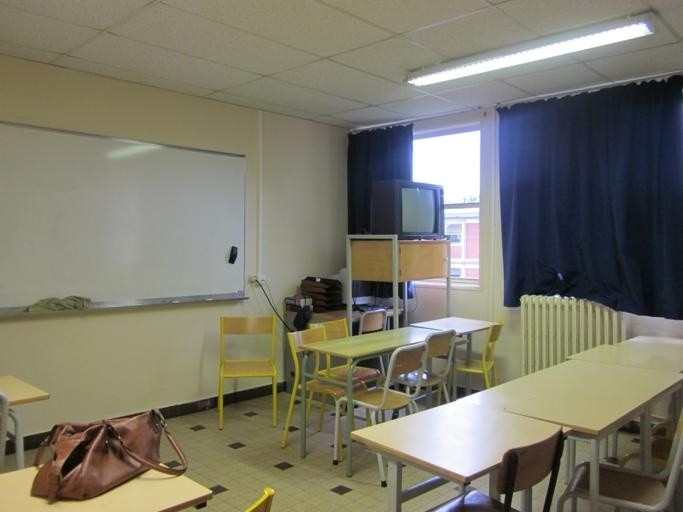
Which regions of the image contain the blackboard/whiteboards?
[0,120,246,318]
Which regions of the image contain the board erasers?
[229,246,237,264]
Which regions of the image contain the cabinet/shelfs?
[345,234,451,334]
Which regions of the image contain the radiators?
[519,294,625,375]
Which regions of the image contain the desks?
[0,374,213,511]
[282,306,409,416]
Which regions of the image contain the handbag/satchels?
[31,407,187,502]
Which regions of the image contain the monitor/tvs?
[368,179,445,240]
